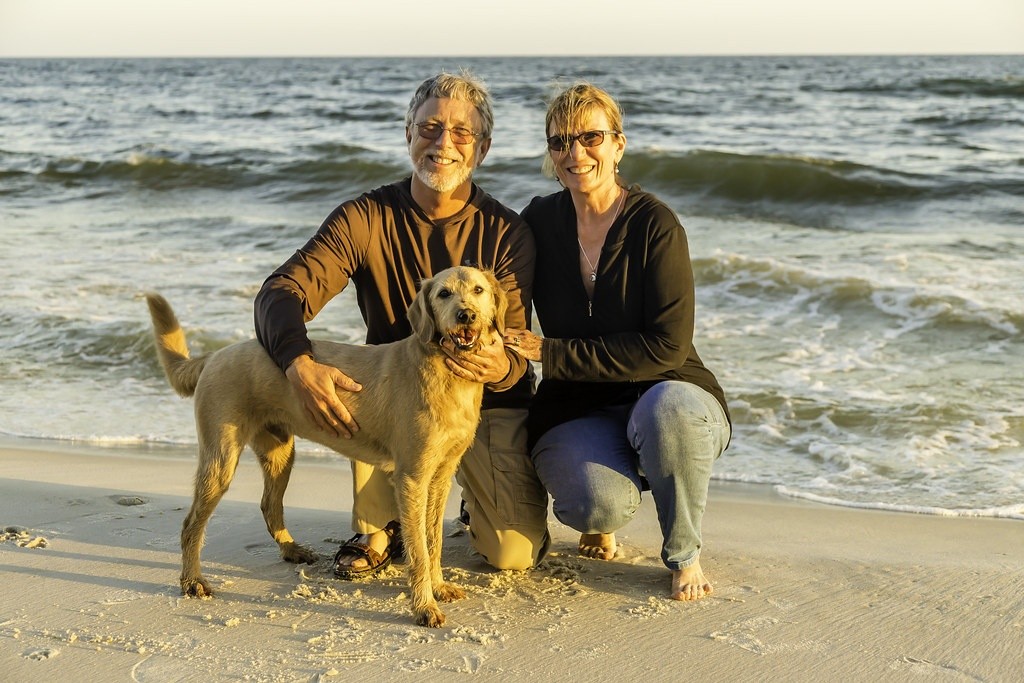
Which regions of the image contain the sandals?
[460,499,470,525]
[335,521,405,580]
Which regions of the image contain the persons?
[254,70,551,579]
[502,83,733,604]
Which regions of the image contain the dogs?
[144,265,509,630]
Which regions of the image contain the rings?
[514,336,520,344]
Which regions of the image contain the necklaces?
[578,189,624,282]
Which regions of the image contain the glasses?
[410,122,483,144]
[547,130,622,152]
